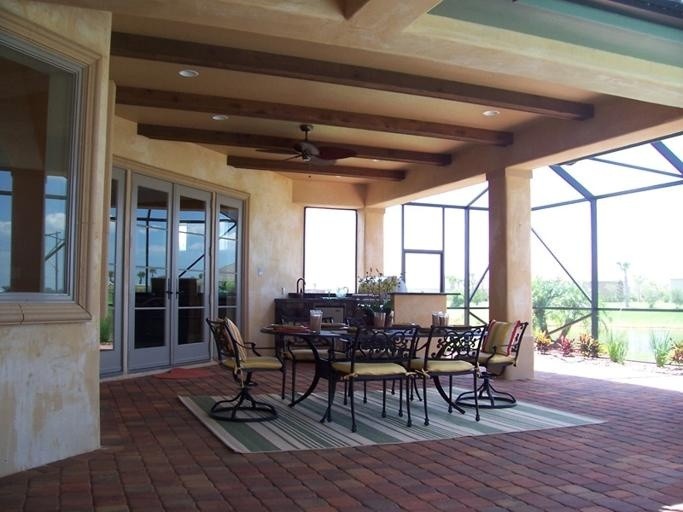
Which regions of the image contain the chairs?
[282,315,349,399]
[344,317,419,404]
[455,319,528,410]
[206,317,289,423]
[319,324,421,432]
[399,324,488,425]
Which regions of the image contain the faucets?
[297,278,305,293]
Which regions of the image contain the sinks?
[288,293,336,298]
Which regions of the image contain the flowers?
[534,329,601,359]
[357,267,406,313]
[668,337,682,367]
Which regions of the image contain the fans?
[255,124,357,163]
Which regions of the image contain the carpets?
[152,368,213,379]
[177,385,608,454]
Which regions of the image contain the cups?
[309,309,324,335]
[336,287,349,298]
